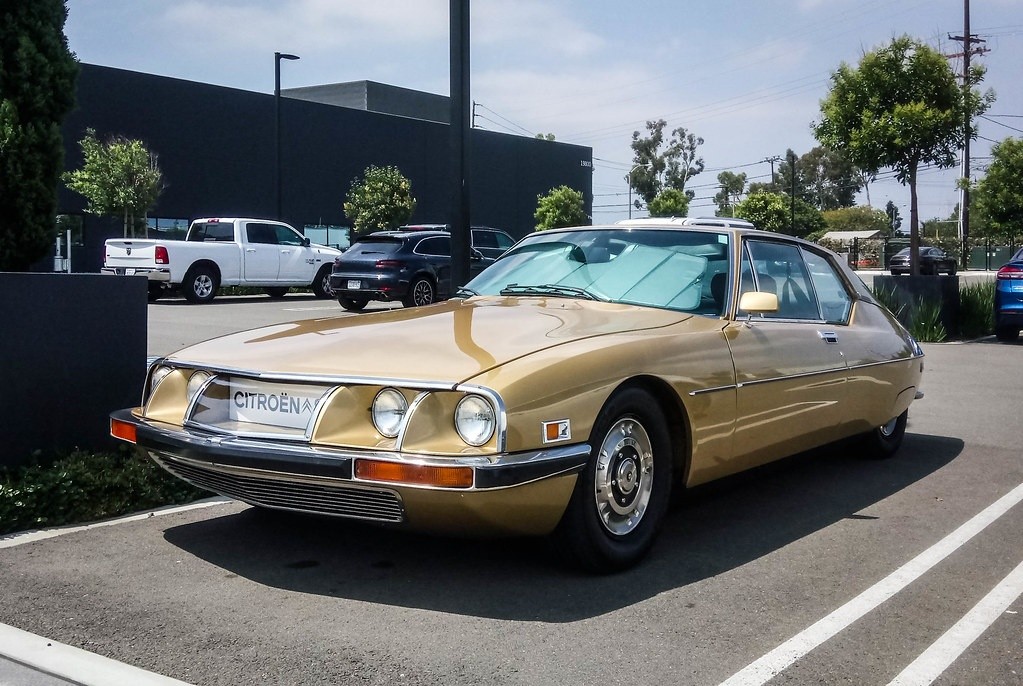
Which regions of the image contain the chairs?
[711,268,780,316]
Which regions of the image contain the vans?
[611,216,756,263]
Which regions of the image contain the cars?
[110,221,928,570]
[888,246,958,275]
[993,248,1023,337]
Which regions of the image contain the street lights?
[273,51,302,218]
[893,204,908,238]
[628,164,650,219]
[719,183,735,218]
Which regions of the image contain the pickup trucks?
[101,217,343,304]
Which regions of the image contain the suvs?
[328,224,495,310]
[468,226,517,258]
